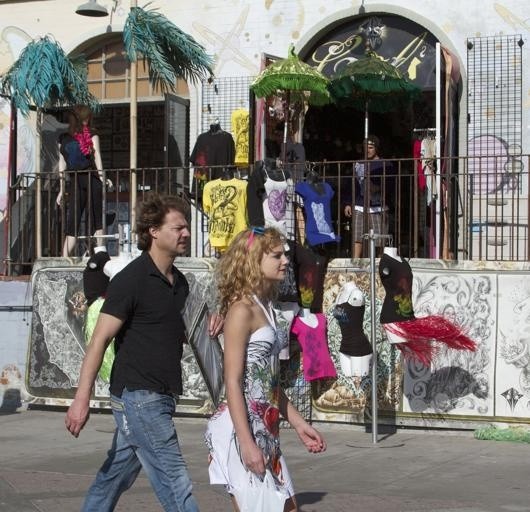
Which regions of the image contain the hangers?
[418,127,436,140]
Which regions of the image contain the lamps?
[75,0,118,17]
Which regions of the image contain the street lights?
[74,0,139,248]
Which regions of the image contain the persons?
[291,307,337,381]
[337,273,351,287]
[64,194,224,512]
[333,281,373,377]
[83,246,115,382]
[439,304,455,323]
[102,252,138,279]
[191,120,235,204]
[58,106,114,258]
[379,246,417,345]
[341,136,394,258]
[203,227,327,512]
